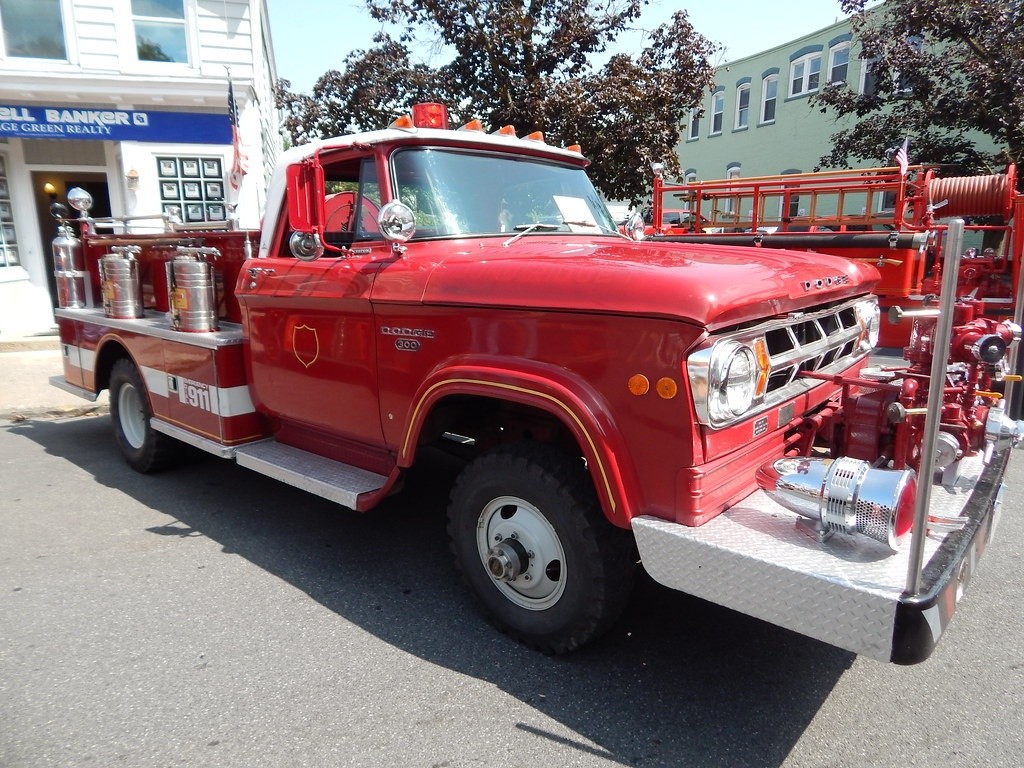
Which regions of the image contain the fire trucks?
[54,101,1024,668]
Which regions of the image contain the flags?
[894,137,910,176]
[227,77,249,192]
